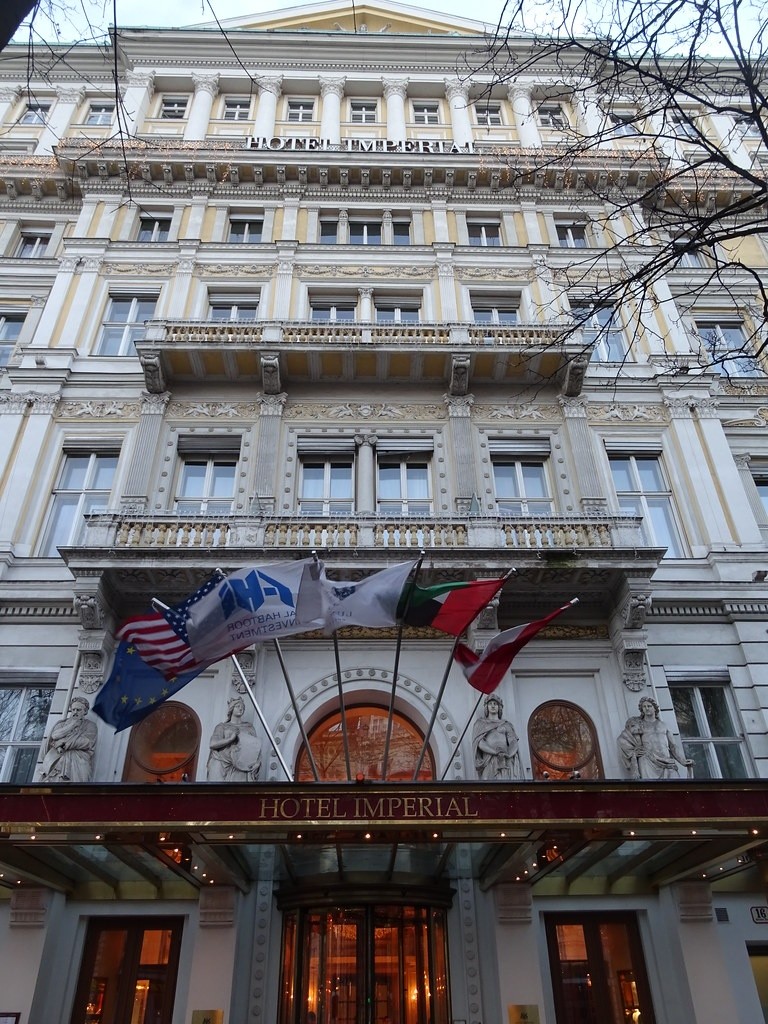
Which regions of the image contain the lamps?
[332,922,392,940]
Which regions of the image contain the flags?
[90,607,209,736]
[295,559,419,635]
[113,574,256,684]
[451,602,572,696]
[397,577,507,638]
[186,557,330,661]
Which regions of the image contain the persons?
[471,693,524,780]
[208,697,261,782]
[617,696,696,778]
[38,696,98,782]
